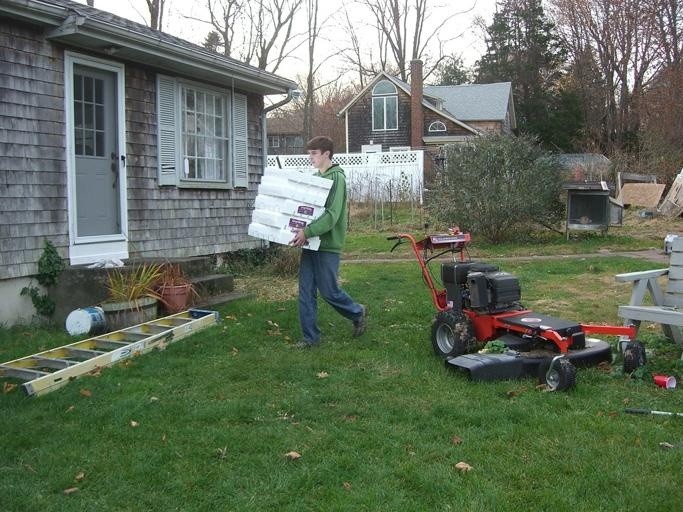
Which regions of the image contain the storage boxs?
[248,165,334,252]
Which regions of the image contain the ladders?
[0,309,219,396]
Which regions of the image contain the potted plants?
[96,257,166,332]
[159,255,204,315]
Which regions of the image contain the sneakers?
[297,340,322,351]
[352,303,366,338]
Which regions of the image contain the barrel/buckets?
[64,306,106,337]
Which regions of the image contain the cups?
[653,375,676,389]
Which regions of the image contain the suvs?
[540,153,612,183]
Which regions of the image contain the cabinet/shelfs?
[560,179,613,242]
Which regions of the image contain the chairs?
[613,234,682,352]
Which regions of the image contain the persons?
[286,137,368,350]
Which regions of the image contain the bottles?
[448,229,454,236]
